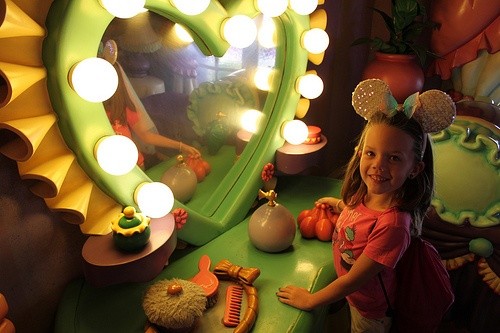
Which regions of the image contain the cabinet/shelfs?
[56,175,346,333]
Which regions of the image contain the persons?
[102,57,202,171]
[275,109,434,333]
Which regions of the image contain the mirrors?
[0,0,329,246]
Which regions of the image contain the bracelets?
[335,200,342,213]
[178,141,182,152]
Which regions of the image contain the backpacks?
[370,207,455,333]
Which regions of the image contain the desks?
[128,76,164,98]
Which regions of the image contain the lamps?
[116,24,162,76]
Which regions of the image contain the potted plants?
[350,0,446,104]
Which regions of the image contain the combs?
[189,254,218,305]
[223,285,243,327]
